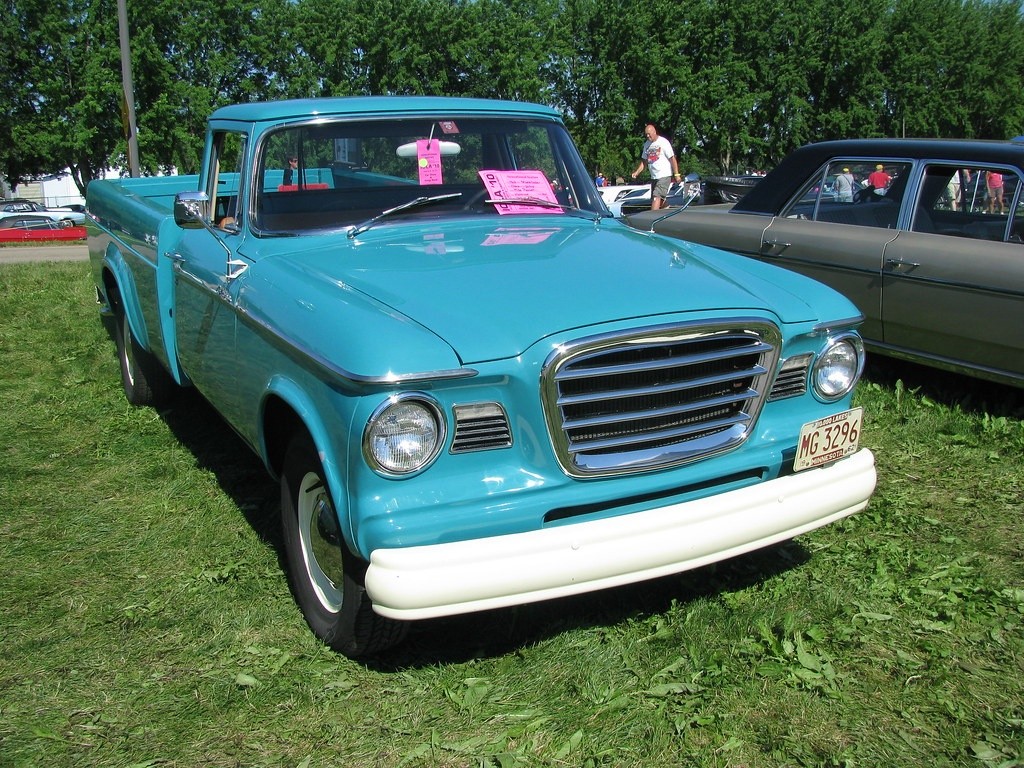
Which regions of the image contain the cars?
[593,180,706,217]
[615,137,1024,400]
[0,197,88,243]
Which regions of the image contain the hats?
[843,167,849,172]
[598,172,602,176]
[876,165,883,170]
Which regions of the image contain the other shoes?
[1000,211,1004,215]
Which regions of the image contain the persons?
[812,164,890,202]
[749,168,767,177]
[596,172,626,187]
[983,171,1005,215]
[553,178,574,206]
[632,124,681,210]
[283,155,298,186]
[945,169,971,211]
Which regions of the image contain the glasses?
[292,160,298,163]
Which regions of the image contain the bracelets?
[673,173,681,177]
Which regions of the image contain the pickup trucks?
[81,93,877,663]
